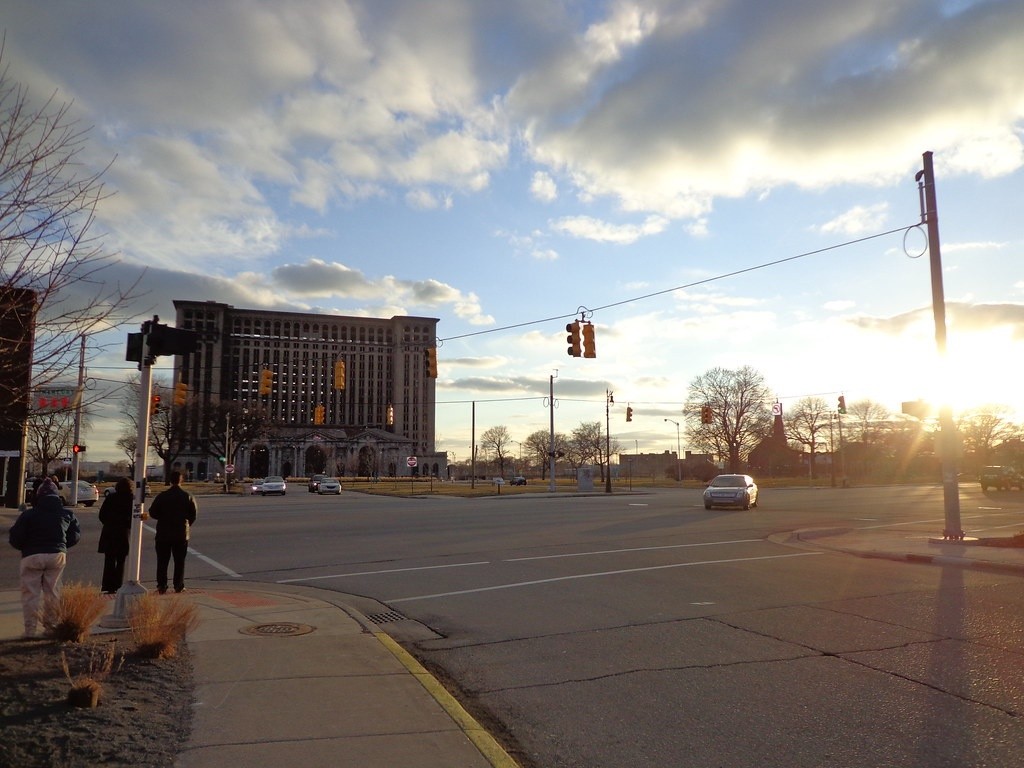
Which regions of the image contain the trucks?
[493,477,505,485]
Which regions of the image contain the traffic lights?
[151,395,161,414]
[73,446,86,452]
[702,407,712,424]
[425,348,437,379]
[566,322,581,357]
[627,407,633,421]
[548,452,554,457]
[838,396,847,414]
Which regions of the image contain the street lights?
[664,418,682,480]
[605,389,615,493]
[511,440,521,459]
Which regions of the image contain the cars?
[249,479,265,495]
[318,478,342,495]
[262,476,287,496]
[25,477,152,507]
[703,474,760,511]
[1013,473,1024,490]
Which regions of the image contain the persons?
[52,475,58,488]
[149,471,197,595]
[98,478,134,593]
[32,475,43,491]
[8,478,80,639]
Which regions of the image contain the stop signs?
[407,457,417,467]
[225,465,234,473]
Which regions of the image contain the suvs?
[980,465,1015,491]
[510,477,527,486]
[308,475,331,493]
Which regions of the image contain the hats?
[37,477,59,496]
